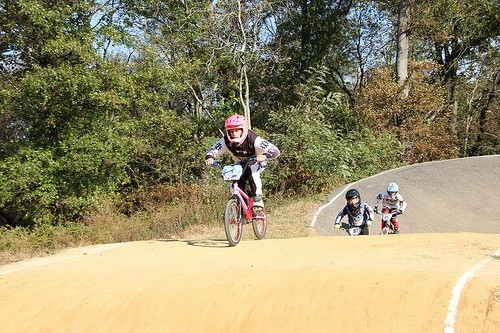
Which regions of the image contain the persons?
[206,114,280,225]
[334,189,374,235]
[374,182,408,234]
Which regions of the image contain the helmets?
[224,114,248,147]
[345,189,361,212]
[387,183,399,199]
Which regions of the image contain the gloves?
[335,224,340,229]
[367,220,372,227]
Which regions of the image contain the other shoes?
[394,228,399,234]
[253,206,263,217]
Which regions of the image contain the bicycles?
[204,156,267,246]
[334,217,374,237]
[376,209,403,236]
